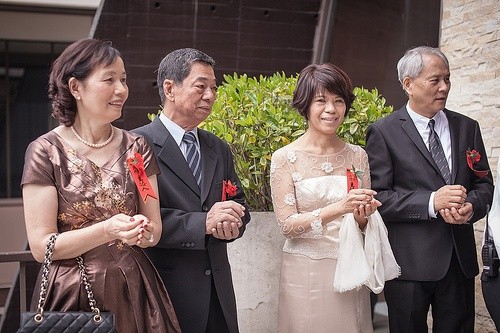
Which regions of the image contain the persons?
[128,47,251,333]
[480,160,500,333]
[270,63,382,333]
[20,39,182,333]
[365,46,495,333]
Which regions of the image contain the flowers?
[288,153,297,163]
[309,219,324,234]
[467,147,481,163]
[225,179,238,197]
[284,193,296,206]
[350,165,365,180]
[312,208,322,218]
[270,159,277,173]
[321,162,334,174]
[291,172,303,182]
[126,152,145,180]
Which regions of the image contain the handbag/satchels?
[19,233,116,332]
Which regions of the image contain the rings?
[147,233,154,242]
[364,216,369,218]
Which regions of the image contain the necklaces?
[70,122,115,148]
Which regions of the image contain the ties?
[182,132,202,189]
[428,119,452,185]
